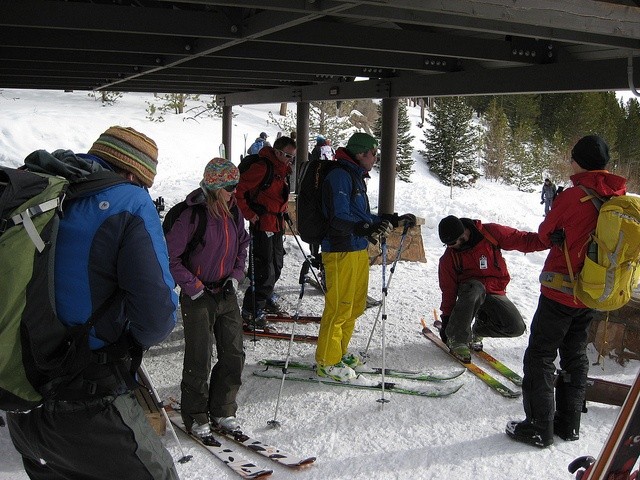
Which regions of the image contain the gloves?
[223,281,236,297]
[383,213,416,230]
[546,229,567,246]
[440,315,449,344]
[194,287,211,303]
[353,219,388,245]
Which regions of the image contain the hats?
[199,157,240,197]
[438,215,464,244]
[87,125,158,187]
[571,135,610,172]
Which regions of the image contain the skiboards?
[242,314,321,343]
[305,269,381,308]
[253,360,465,397]
[163,396,316,478]
[422,312,523,398]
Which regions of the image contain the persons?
[276,131,282,139]
[537,178,561,218]
[289,130,298,139]
[246,132,272,155]
[238,137,299,336]
[437,214,563,363]
[0,124,180,479]
[312,132,417,382]
[167,157,250,440]
[506,134,640,450]
[321,136,337,152]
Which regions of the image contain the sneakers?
[469,332,483,352]
[190,419,212,438]
[316,361,355,383]
[448,339,471,364]
[213,415,240,432]
[343,353,368,372]
[554,423,580,442]
[264,297,285,313]
[241,313,269,328]
[506,421,553,448]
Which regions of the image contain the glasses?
[447,242,457,246]
[223,183,240,192]
[371,151,377,157]
[284,153,295,159]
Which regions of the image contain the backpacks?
[296,159,358,245]
[163,200,207,264]
[1,149,136,414]
[562,186,640,312]
[238,154,290,196]
[251,142,263,154]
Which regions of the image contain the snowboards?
[552,369,631,404]
[569,370,640,480]
